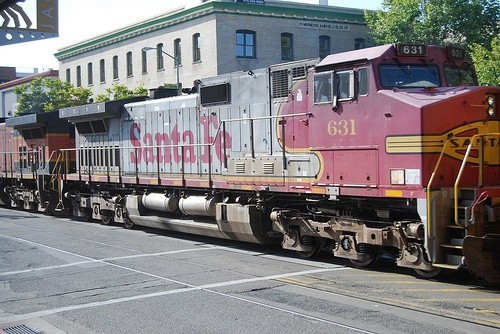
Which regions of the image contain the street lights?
[142,46,179,84]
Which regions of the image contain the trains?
[0,42,500,290]
